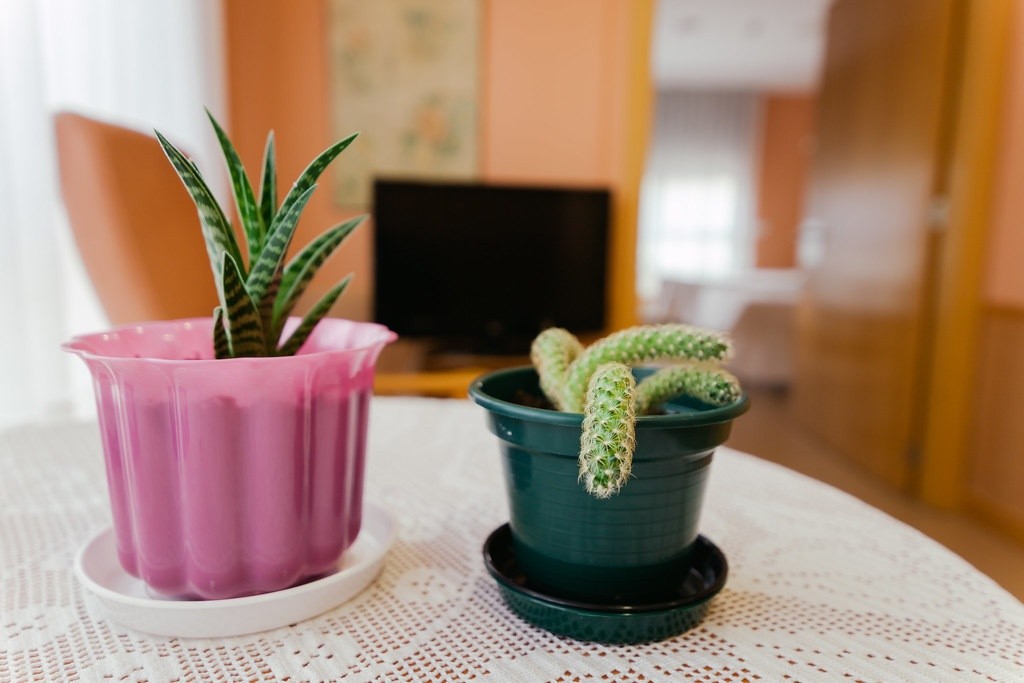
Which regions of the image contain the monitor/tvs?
[369,176,611,339]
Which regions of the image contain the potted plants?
[53,109,405,643]
[467,326,751,645]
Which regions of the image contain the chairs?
[57,110,215,325]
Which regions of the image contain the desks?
[3,394,1024,683]
